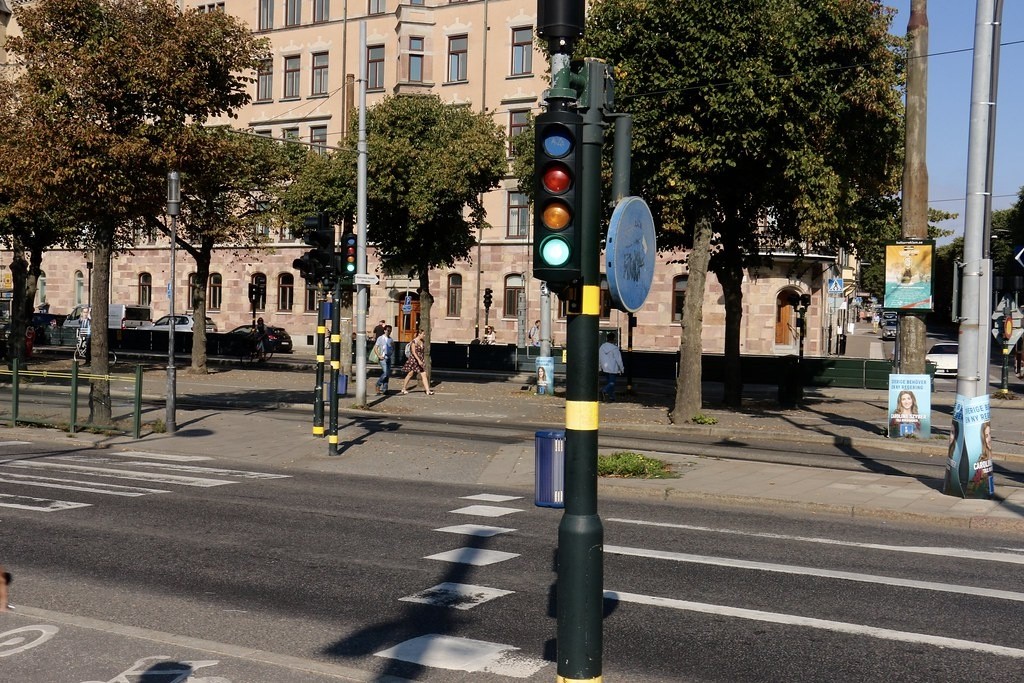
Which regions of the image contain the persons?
[947,420,992,487]
[530,320,540,346]
[326,328,331,349]
[891,391,921,431]
[375,325,395,395]
[488,325,495,341]
[79,335,91,367]
[866,304,900,360]
[599,333,624,402]
[401,329,434,396]
[373,320,385,336]
[248,317,269,361]
[353,332,357,339]
[79,307,91,328]
[538,366,546,382]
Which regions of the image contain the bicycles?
[237,332,275,365]
[74,336,118,367]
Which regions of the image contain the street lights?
[164,170,183,434]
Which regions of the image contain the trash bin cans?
[599,326,621,351]
[837,334,847,355]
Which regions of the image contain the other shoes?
[599,392,603,401]
[375,385,380,394]
[258,359,261,362]
[83,362,90,367]
[607,398,615,403]
[262,359,265,362]
[426,391,434,395]
[402,388,409,395]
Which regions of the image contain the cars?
[865,302,899,324]
[924,343,959,379]
[881,318,900,340]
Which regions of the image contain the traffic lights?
[484,288,493,307]
[341,232,357,276]
[532,109,584,283]
[292,254,320,280]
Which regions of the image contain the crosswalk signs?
[826,279,843,293]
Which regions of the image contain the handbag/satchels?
[405,339,416,358]
[529,327,536,339]
[369,345,381,363]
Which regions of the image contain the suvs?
[133,313,218,330]
[224,325,294,354]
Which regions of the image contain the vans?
[58,302,155,346]
[0,313,66,352]
